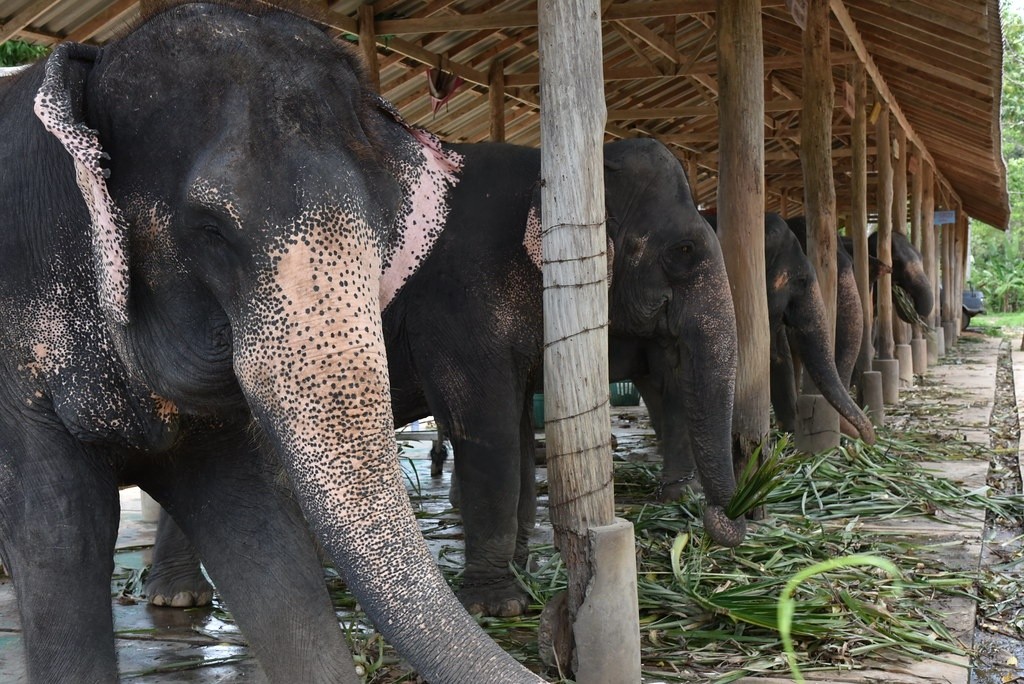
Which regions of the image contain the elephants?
[0,0,935,684]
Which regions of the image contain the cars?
[939,288,984,329]
